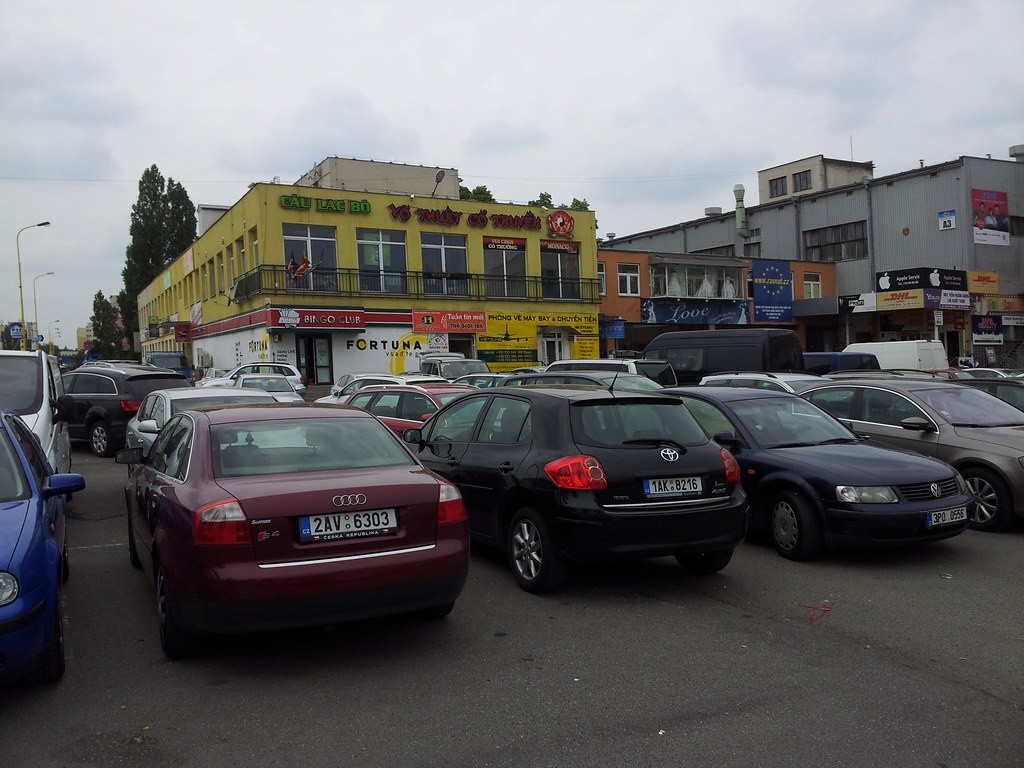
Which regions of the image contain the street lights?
[16,221,51,351]
[32,271,55,351]
[48,319,61,352]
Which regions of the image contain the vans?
[143,350,196,388]
[840,339,951,377]
[637,329,805,388]
[802,351,881,377]
[0,349,79,509]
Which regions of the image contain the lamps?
[431,170,445,197]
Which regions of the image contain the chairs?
[375,398,428,420]
[490,407,530,444]
[691,348,705,371]
[222,444,269,470]
[298,426,351,464]
[266,382,278,391]
[733,413,779,444]
[810,398,851,420]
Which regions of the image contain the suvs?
[194,361,307,396]
[61,359,194,459]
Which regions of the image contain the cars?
[653,386,976,563]
[403,385,748,595]
[0,406,85,691]
[234,374,305,404]
[776,379,1023,533]
[125,388,314,478]
[929,367,1023,414]
[698,371,853,402]
[202,367,247,379]
[315,350,680,441]
[114,402,469,658]
